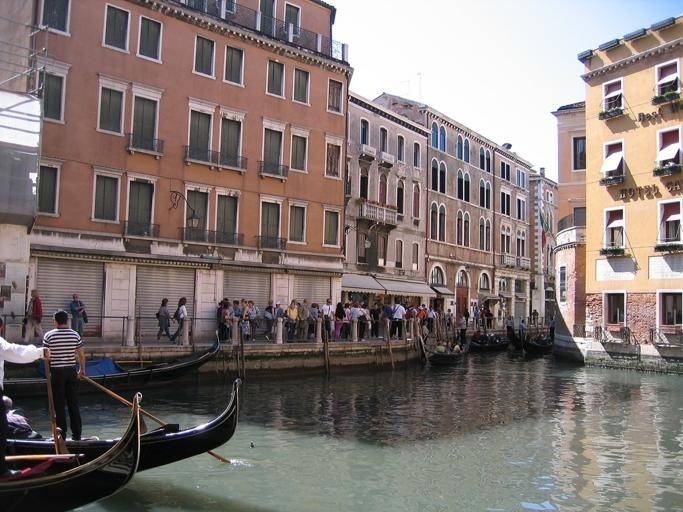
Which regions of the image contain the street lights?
[545,286,554,319]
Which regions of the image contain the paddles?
[78,374,232,463]
[42,346,71,455]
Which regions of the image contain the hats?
[418,306,423,310]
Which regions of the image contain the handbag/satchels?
[83,312,88,323]
[264,310,273,320]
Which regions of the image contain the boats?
[5,377,242,474]
[2,329,220,397]
[524,338,552,354]
[507,326,528,349]
[0,392,143,511]
[418,335,449,365]
[469,339,510,350]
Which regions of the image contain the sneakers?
[0,469,21,480]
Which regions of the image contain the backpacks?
[174,306,183,320]
[405,308,413,319]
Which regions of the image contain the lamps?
[343,224,374,251]
[377,254,384,266]
[408,263,419,273]
[169,190,199,227]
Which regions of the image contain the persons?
[157,297,187,343]
[464,307,493,329]
[264,297,454,342]
[472,330,500,344]
[70,293,87,341]
[0,317,51,480]
[217,296,260,342]
[1,396,43,441]
[434,315,468,353]
[506,310,554,345]
[22,289,44,343]
[666,309,681,324]
[43,310,87,442]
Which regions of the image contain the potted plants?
[595,91,683,257]
